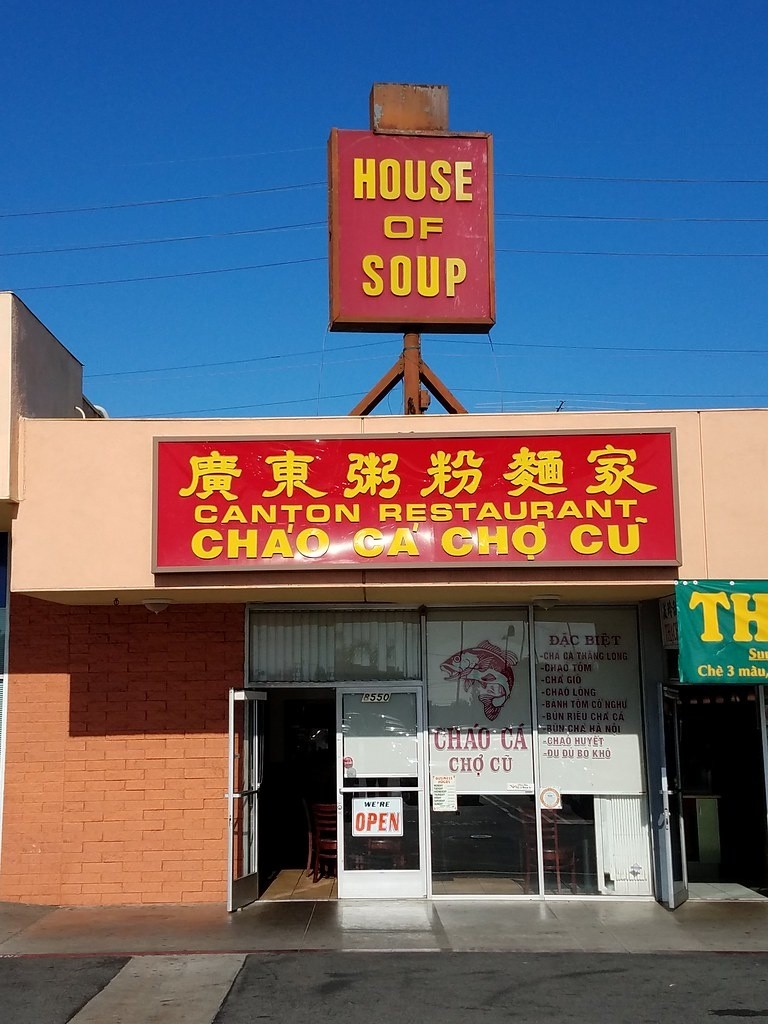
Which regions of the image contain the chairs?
[304,802,438,884]
[522,806,578,895]
